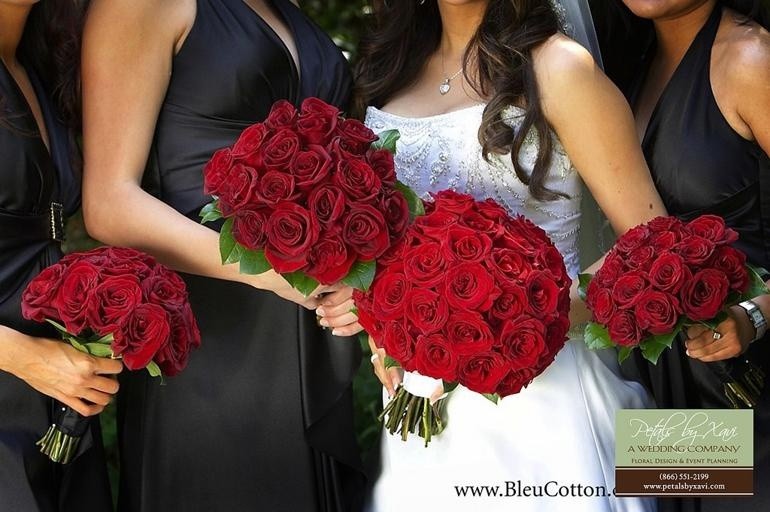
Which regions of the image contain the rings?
[712,329,721,341]
[370,354,378,363]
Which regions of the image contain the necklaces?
[435,20,475,96]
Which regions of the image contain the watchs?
[740,299,766,345]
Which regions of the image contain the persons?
[356,1,674,512]
[581,0,769,511]
[1,0,127,512]
[78,0,388,512]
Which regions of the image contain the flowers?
[578,215,770,411]
[20,246,201,464]
[338,188,573,447]
[200,96,425,295]
